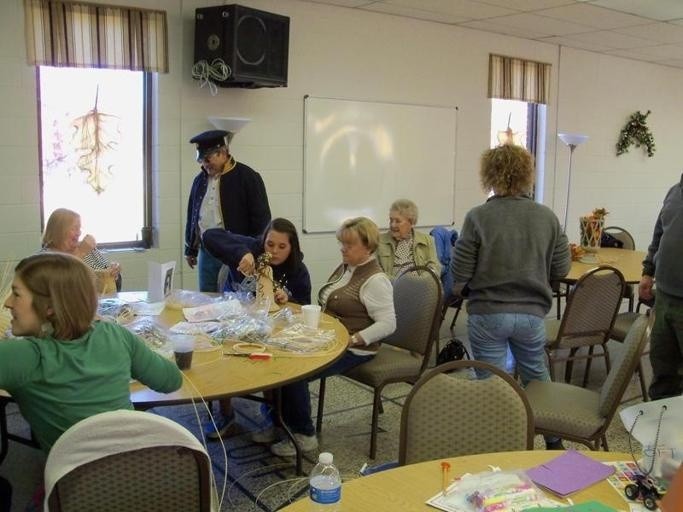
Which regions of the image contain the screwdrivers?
[224,351,272,360]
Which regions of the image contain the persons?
[250,216,398,457]
[637,172,683,399]
[201,216,312,439]
[32,204,123,284]
[1,250,184,458]
[449,143,573,451]
[375,199,443,278]
[184,127,273,295]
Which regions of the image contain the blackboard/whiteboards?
[297,95,458,234]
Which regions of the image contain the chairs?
[42,409,213,512]
[523,309,656,450]
[428,228,471,328]
[315,267,443,459]
[582,311,652,402]
[544,263,625,388]
[635,289,657,315]
[604,227,636,312]
[398,360,536,464]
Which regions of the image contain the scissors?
[237,265,260,280]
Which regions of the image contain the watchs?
[351,333,359,345]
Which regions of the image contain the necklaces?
[347,265,354,273]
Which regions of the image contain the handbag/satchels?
[437,339,470,374]
[619,395,683,479]
[601,230,624,247]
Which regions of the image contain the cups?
[170,335,194,369]
[302,305,321,329]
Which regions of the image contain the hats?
[190,131,227,149]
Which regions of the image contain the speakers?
[192,4,290,90]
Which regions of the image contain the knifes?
[224,353,271,360]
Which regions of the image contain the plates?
[233,343,265,353]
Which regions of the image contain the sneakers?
[252,427,274,443]
[205,413,234,438]
[271,433,318,456]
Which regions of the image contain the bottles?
[309,452,342,512]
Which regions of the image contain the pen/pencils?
[466,483,541,512]
[441,461,450,497]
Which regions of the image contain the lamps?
[210,116,251,157]
[556,131,587,233]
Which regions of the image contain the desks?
[0,288,350,473]
[556,246,655,320]
[279,450,683,512]
